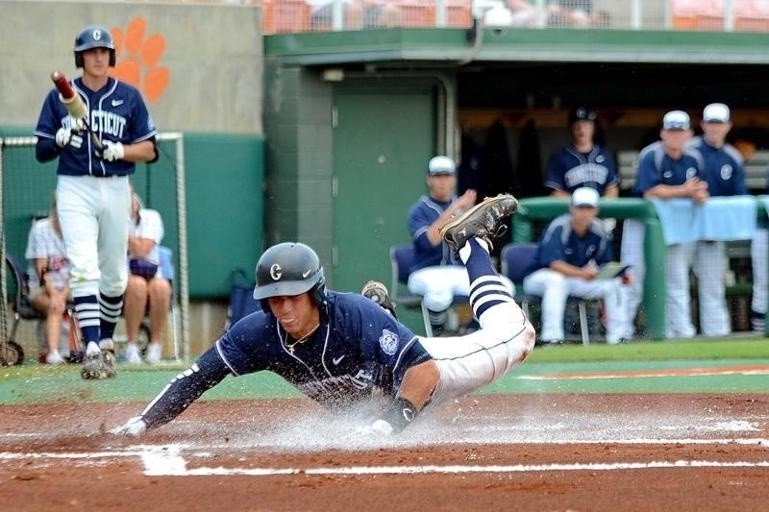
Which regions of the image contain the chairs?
[6,256,86,359]
[387,245,468,338]
[499,243,598,344]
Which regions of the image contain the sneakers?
[362,280,395,319]
[46,353,66,364]
[124,344,142,364]
[144,343,163,365]
[81,341,106,379]
[437,193,518,253]
[100,338,116,375]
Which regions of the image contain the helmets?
[73,27,116,68]
[568,105,598,122]
[253,242,329,313]
[131,257,157,278]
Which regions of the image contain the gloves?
[347,421,396,449]
[56,127,84,153]
[102,416,147,437]
[96,140,124,163]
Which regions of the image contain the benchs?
[617,152,768,190]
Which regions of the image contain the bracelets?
[379,395,418,436]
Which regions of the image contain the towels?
[649,196,757,247]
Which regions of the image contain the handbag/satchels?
[224,268,262,335]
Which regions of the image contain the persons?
[545,0,611,30]
[23,198,72,365]
[408,154,517,338]
[619,110,709,340]
[304,0,402,30]
[123,185,171,365]
[34,27,159,381]
[544,111,619,198]
[522,186,635,349]
[750,228,768,332]
[106,193,536,440]
[687,103,750,335]
[471,1,548,29]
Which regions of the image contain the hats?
[428,155,457,177]
[571,187,600,208]
[662,110,691,131]
[702,103,732,124]
